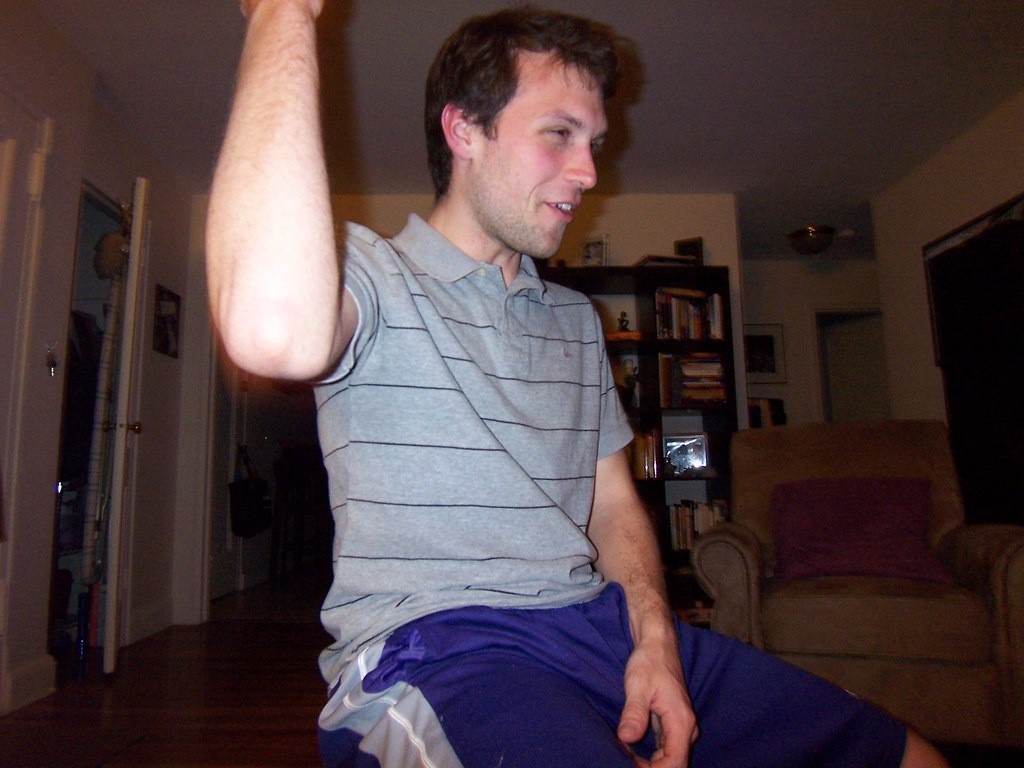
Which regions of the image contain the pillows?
[772,477,955,584]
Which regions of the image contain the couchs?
[692,420,1024,747]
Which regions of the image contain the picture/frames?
[153,283,181,358]
[576,231,610,268]
[744,323,787,383]
[662,433,710,471]
[675,236,703,258]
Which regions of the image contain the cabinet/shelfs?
[538,264,739,580]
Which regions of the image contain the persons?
[205,0,948,768]
[676,444,704,467]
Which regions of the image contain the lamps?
[789,225,835,255]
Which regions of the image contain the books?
[622,426,657,479]
[671,499,727,550]
[656,291,726,409]
